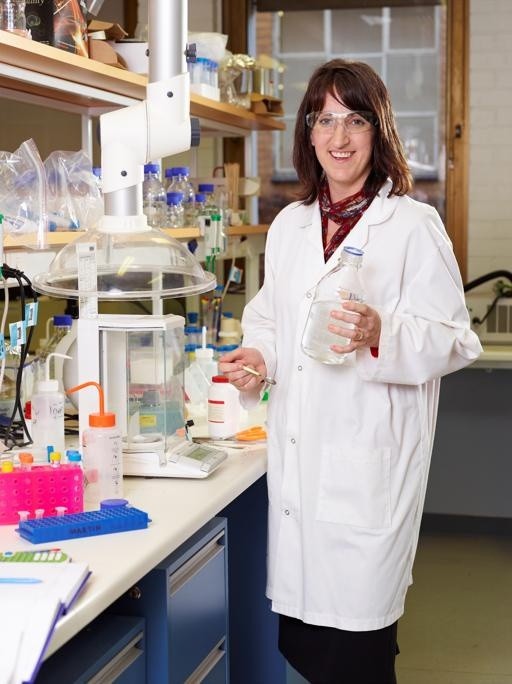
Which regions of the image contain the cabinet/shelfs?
[148,519,233,683]
[60,616,148,683]
[0,28,285,444]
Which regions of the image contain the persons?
[219,58,485,684]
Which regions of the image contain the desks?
[424,332,512,521]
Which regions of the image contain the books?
[0,561,92,684]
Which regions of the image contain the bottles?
[303,247,366,367]
[2,162,239,233]
[190,58,219,87]
[0,301,239,511]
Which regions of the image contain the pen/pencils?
[0,578,44,585]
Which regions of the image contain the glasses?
[306,111,375,135]
